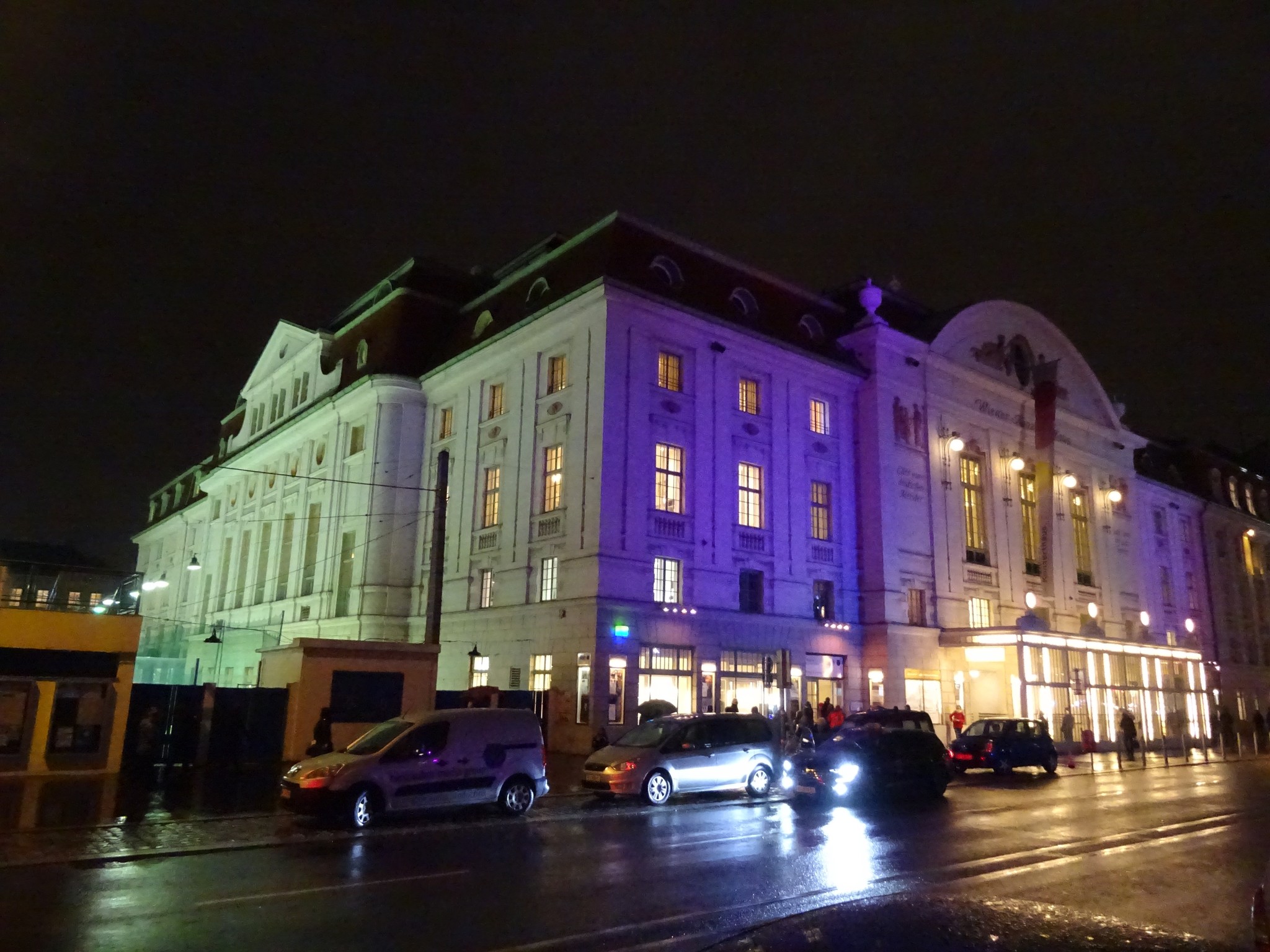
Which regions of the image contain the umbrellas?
[636,699,679,718]
[458,685,499,707]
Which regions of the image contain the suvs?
[579,712,779,805]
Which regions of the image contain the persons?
[313,706,335,756]
[721,694,1270,766]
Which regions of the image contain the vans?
[280,709,549,829]
[839,709,938,734]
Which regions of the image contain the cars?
[944,717,1059,780]
[777,728,955,802]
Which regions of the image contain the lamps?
[1103,487,1122,534]
[947,431,965,490]
[1057,470,1077,520]
[1004,453,1025,506]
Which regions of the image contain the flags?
[1030,362,1057,599]
